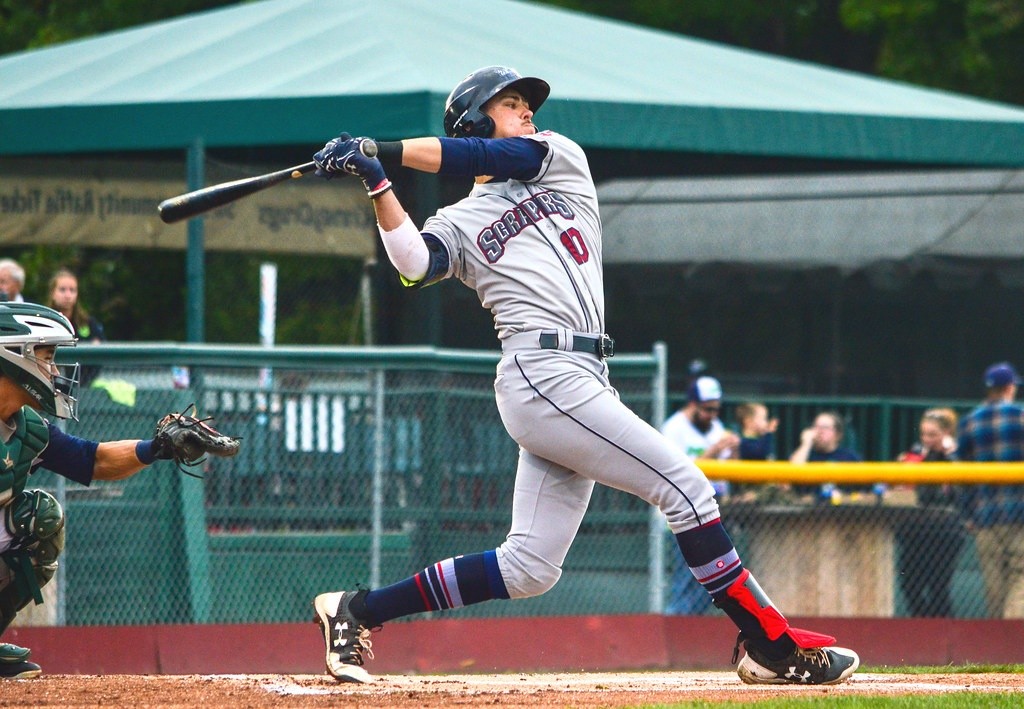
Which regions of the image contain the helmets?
[443,66,551,139]
[0,301,81,423]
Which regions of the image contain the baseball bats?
[156,137,378,225]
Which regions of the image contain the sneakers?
[737,640,859,684]
[311,583,374,684]
[0,643,42,679]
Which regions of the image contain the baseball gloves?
[154,402,249,480]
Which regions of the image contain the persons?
[313,68,859,685]
[0,300,243,680]
[663,365,1024,620]
[0,259,103,345]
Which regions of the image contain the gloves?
[313,131,386,191]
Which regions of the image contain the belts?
[540,334,616,359]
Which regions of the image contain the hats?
[987,363,1024,387]
[687,377,722,402]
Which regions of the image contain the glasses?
[702,405,721,413]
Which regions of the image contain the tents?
[0,0,1024,344]
